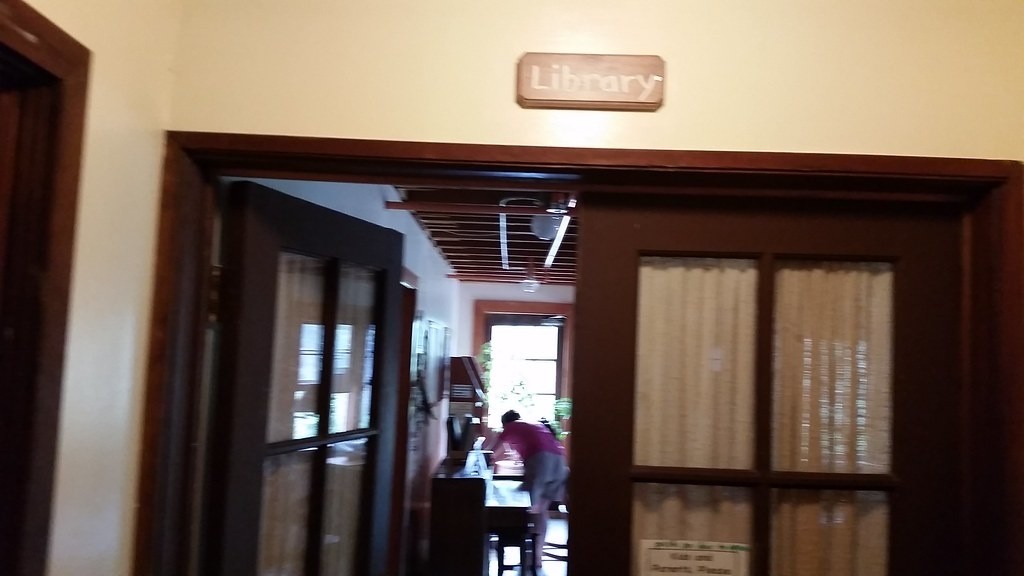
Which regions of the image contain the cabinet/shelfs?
[431,456,533,576]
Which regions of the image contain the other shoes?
[514,566,544,576]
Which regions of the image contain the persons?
[486,410,567,567]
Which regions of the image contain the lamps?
[531,213,563,241]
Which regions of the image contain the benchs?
[497,523,541,576]
